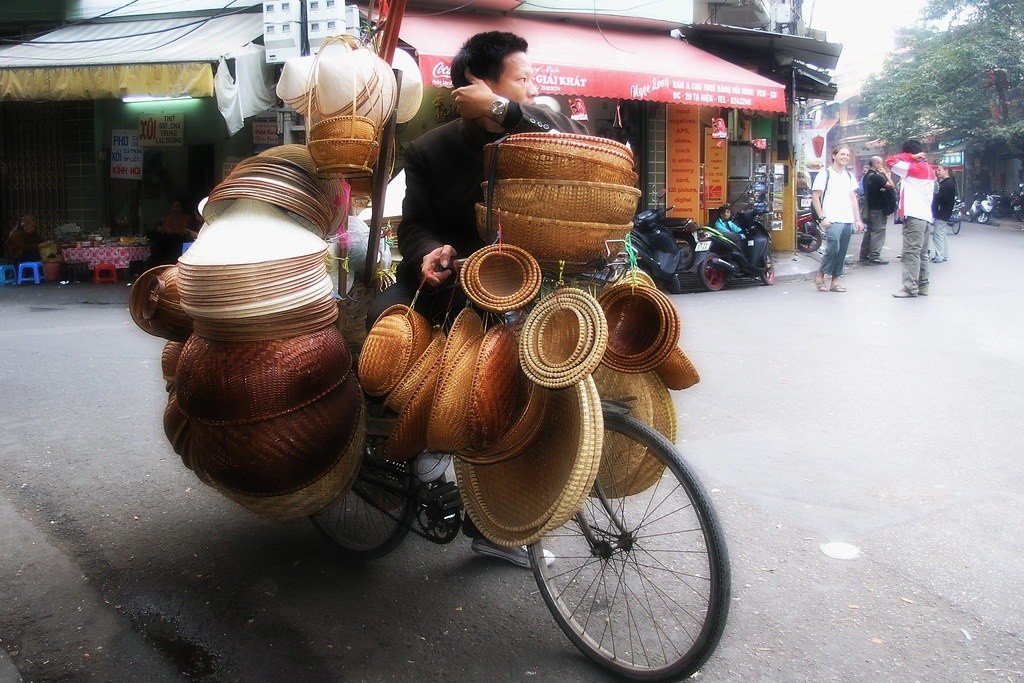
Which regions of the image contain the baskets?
[127,33,701,546]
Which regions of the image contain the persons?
[860,156,956,264]
[714,206,746,240]
[147,199,205,260]
[5,215,43,261]
[365,30,590,568]
[811,145,863,292]
[885,140,935,297]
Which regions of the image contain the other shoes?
[867,257,890,266]
[931,257,948,263]
[859,256,870,264]
[897,253,902,258]
[892,289,927,297]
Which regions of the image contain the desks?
[62,245,154,280]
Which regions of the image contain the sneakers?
[415,448,452,483]
[471,536,556,570]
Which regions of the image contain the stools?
[93,264,118,284]
[17,261,45,284]
[0,264,16,287]
[59,259,91,283]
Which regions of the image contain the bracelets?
[819,217,826,223]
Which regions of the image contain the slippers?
[814,276,829,292]
[829,285,847,292]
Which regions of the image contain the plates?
[99,245,106,247]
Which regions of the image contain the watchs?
[488,97,510,122]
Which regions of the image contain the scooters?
[953,181,1024,225]
[628,187,683,296]
[691,194,775,291]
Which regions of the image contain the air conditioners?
[776,4,794,22]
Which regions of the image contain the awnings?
[0,13,264,97]
[355,3,785,113]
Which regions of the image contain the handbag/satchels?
[860,201,870,224]
[810,196,822,221]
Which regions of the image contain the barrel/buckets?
[44,262,59,281]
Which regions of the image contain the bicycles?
[307,251,732,683]
[947,207,961,236]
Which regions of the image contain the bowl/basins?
[118,241,135,247]
[95,236,102,241]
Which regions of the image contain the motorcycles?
[636,216,696,274]
[796,209,823,252]
